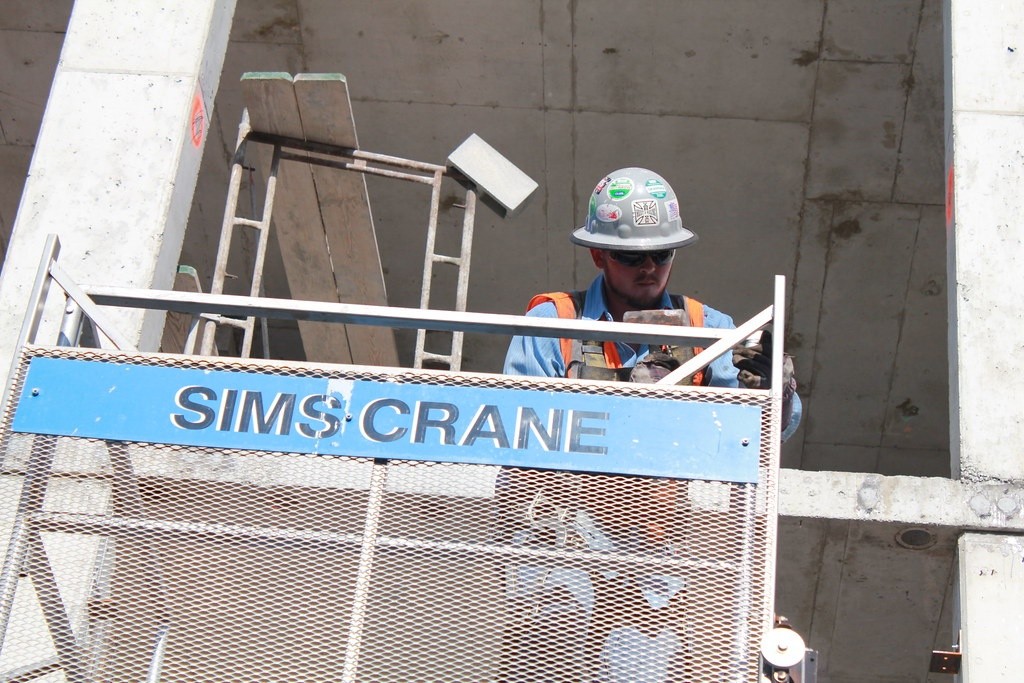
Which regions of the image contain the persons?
[496,166,802,683]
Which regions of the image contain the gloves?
[732,329,797,432]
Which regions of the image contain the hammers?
[623,308,691,354]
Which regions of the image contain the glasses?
[607,247,675,265]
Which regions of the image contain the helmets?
[569,166,700,251]
